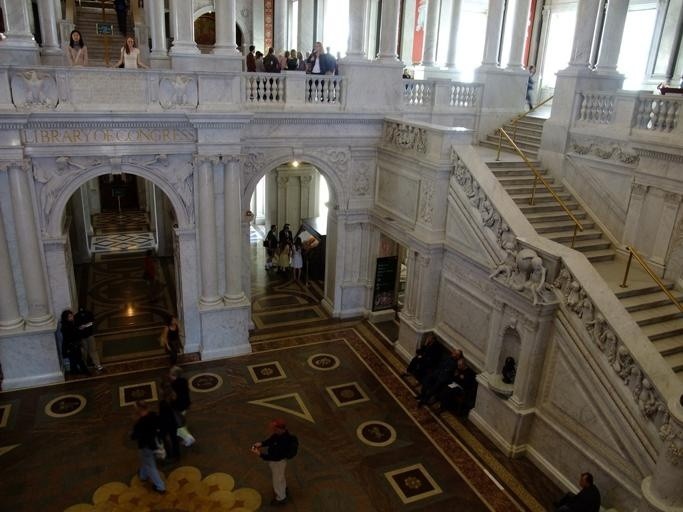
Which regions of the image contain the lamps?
[291,152,299,168]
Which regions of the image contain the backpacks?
[286,436,298,458]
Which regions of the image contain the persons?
[246,42,341,103]
[550,473,600,512]
[403,69,413,90]
[74,304,103,371]
[265,224,303,282]
[400,335,477,417]
[248,418,290,506]
[127,366,196,493]
[67,31,89,67]
[526,66,536,111]
[112,35,150,70]
[163,315,184,368]
[60,310,92,376]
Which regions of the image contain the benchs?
[416,331,478,413]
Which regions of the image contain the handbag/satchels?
[174,411,184,426]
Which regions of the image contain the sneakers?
[271,498,287,504]
